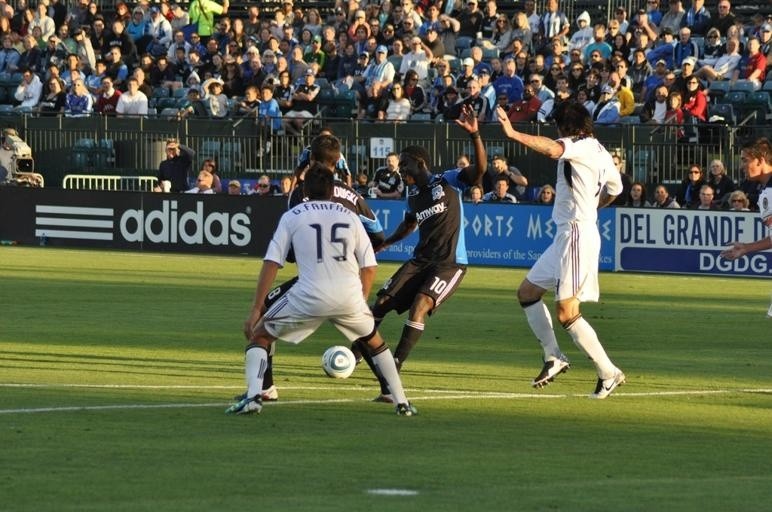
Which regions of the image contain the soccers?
[322,346,356,377]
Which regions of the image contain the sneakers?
[234,383,279,402]
[223,395,263,418]
[531,352,571,389]
[350,341,364,364]
[586,367,626,401]
[375,391,394,404]
[395,401,418,417]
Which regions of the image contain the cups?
[368,187,378,199]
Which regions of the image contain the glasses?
[688,171,702,174]
[258,184,270,189]
[731,199,744,203]
[48,0,731,103]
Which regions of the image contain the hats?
[227,180,241,188]
[760,23,771,33]
[490,153,507,161]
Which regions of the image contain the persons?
[0,0,771,213]
[718,136,772,321]
[256,133,385,402]
[350,103,488,403]
[495,99,626,401]
[223,165,421,417]
